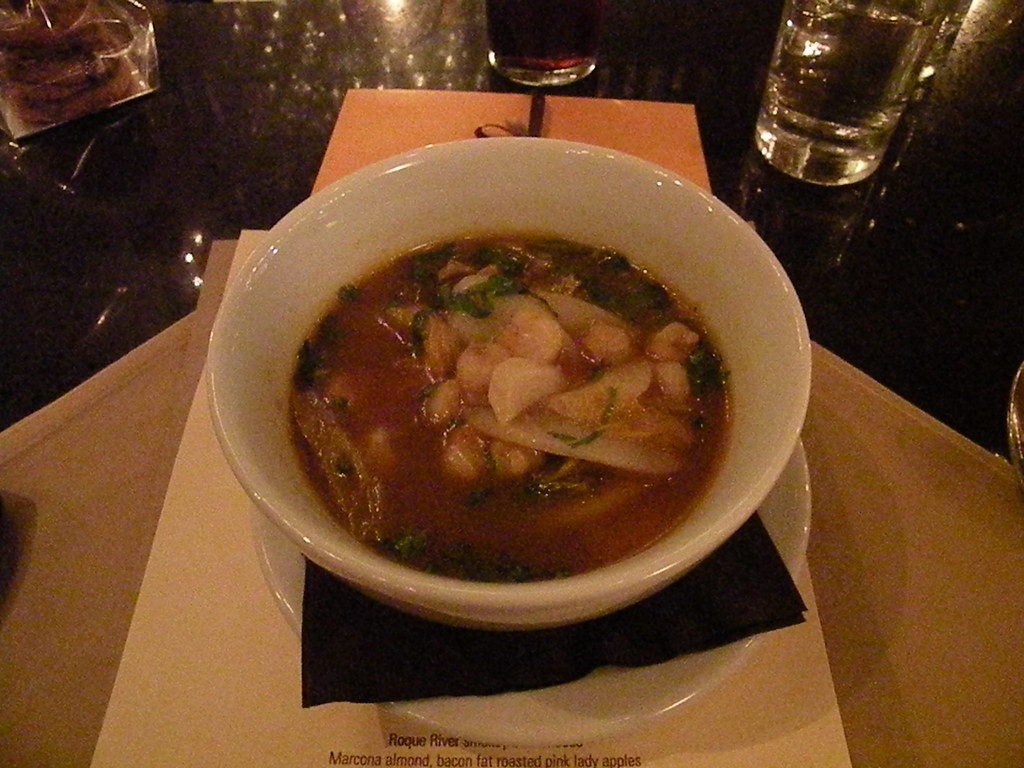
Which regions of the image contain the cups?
[483,0,602,84]
[752,1,974,186]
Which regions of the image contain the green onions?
[293,235,728,583]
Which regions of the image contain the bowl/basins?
[205,136,810,624]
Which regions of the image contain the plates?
[251,437,810,749]
